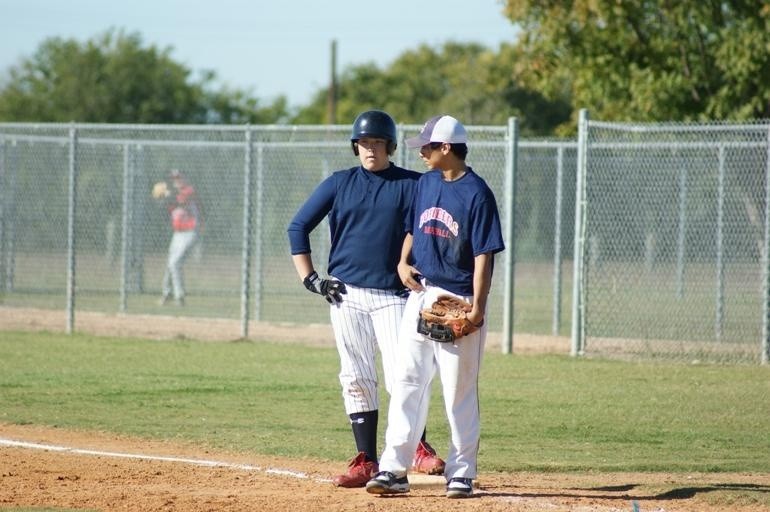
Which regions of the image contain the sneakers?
[447,478,473,498]
[333,452,410,494]
[411,442,445,474]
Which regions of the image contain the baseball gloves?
[417,295,483,342]
[153,182,169,199]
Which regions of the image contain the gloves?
[303,271,345,302]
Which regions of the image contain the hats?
[405,115,466,148]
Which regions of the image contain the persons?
[288,112,447,488]
[152,167,204,306]
[365,114,504,499]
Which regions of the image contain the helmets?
[351,111,397,155]
